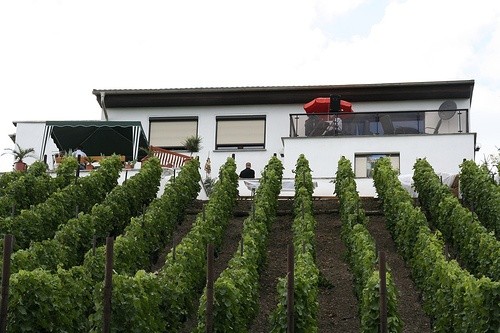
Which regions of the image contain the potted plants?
[84,156,93,170]
[1,144,38,171]
[125,159,138,169]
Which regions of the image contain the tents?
[39,121,149,163]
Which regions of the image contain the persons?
[72,145,88,170]
[239,162,255,178]
[305,111,320,136]
[55,154,63,163]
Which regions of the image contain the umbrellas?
[304,97,353,121]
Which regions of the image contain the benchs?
[55,155,125,169]
